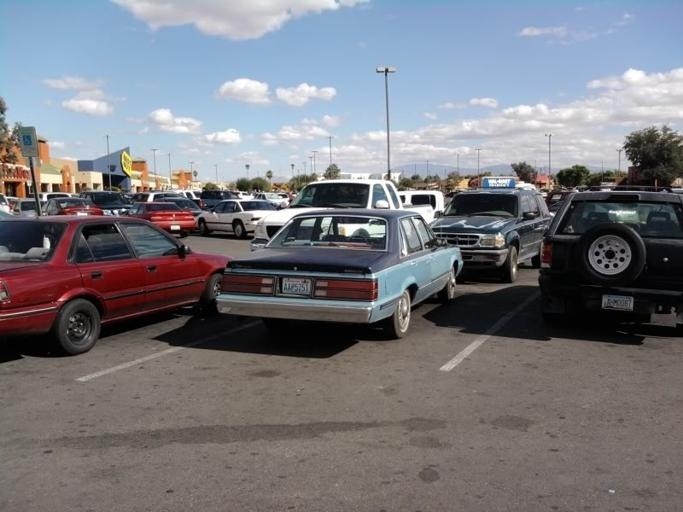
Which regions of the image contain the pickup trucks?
[251,177,435,255]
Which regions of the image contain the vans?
[397,190,446,219]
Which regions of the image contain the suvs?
[431,187,556,282]
[538,192,683,323]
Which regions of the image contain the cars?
[0,187,293,242]
[215,206,470,338]
[0,209,236,356]
[480,176,683,227]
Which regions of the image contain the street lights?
[616,148,623,177]
[544,132,554,178]
[375,66,398,178]
[244,163,249,185]
[413,148,483,179]
[106,134,224,191]
[289,134,335,181]
